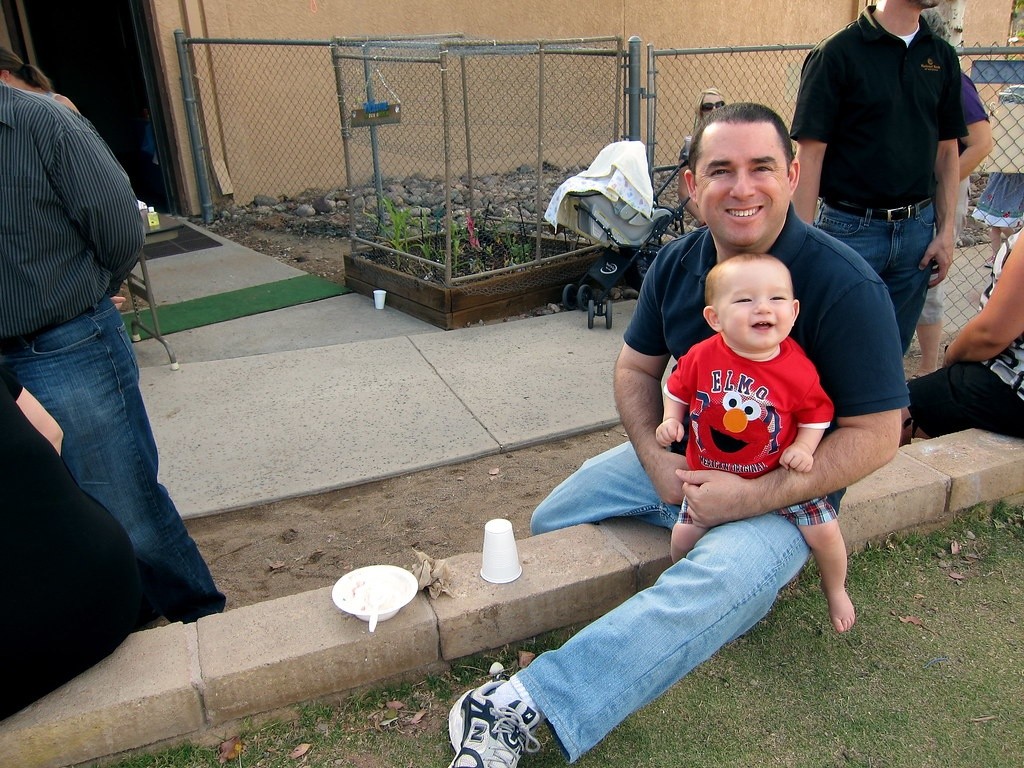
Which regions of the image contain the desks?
[127,211,185,371]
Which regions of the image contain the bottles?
[145,207,159,228]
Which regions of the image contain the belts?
[824,196,932,222]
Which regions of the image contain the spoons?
[365,586,385,632]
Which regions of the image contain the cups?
[373,289,386,310]
[480,519,523,583]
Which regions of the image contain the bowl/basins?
[332,565,419,622]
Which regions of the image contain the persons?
[905,82,1024,441]
[677,88,739,225]
[2,42,228,619]
[789,1,995,357]
[446,102,911,768]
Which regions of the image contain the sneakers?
[449,679,541,768]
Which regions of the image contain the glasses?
[700,101,725,112]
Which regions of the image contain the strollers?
[543,139,697,331]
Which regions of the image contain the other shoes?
[984,254,996,267]
